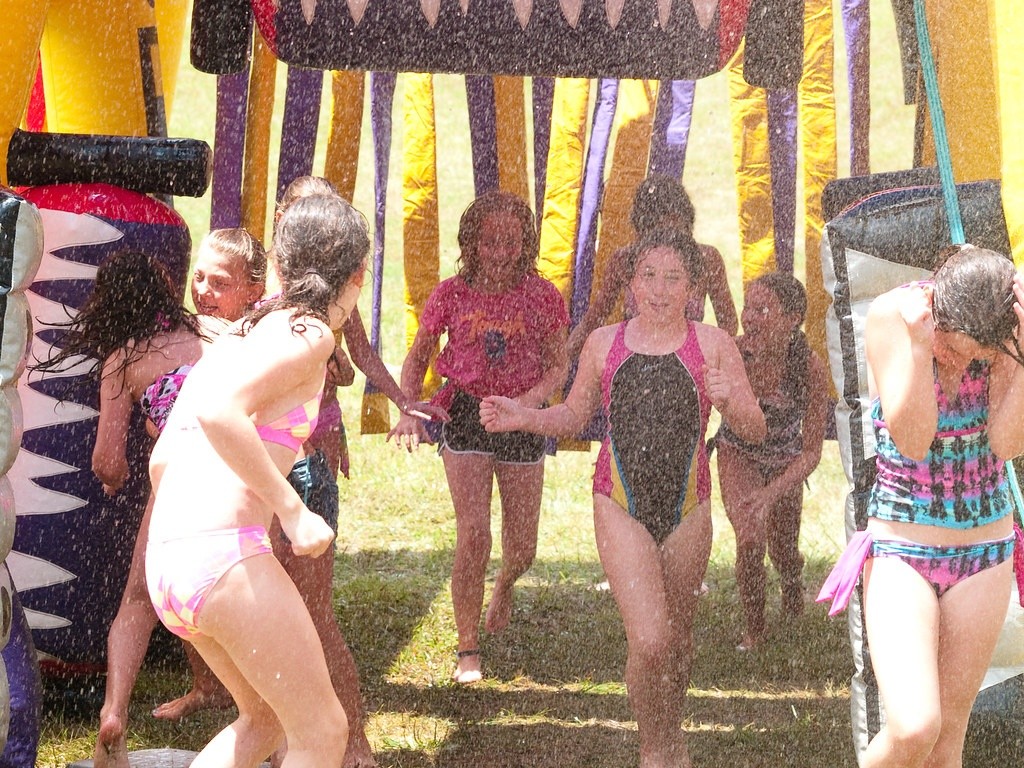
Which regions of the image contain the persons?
[28,177,818,767]
[143,193,371,768]
[814,242,1024,768]
[477,228,766,767]
